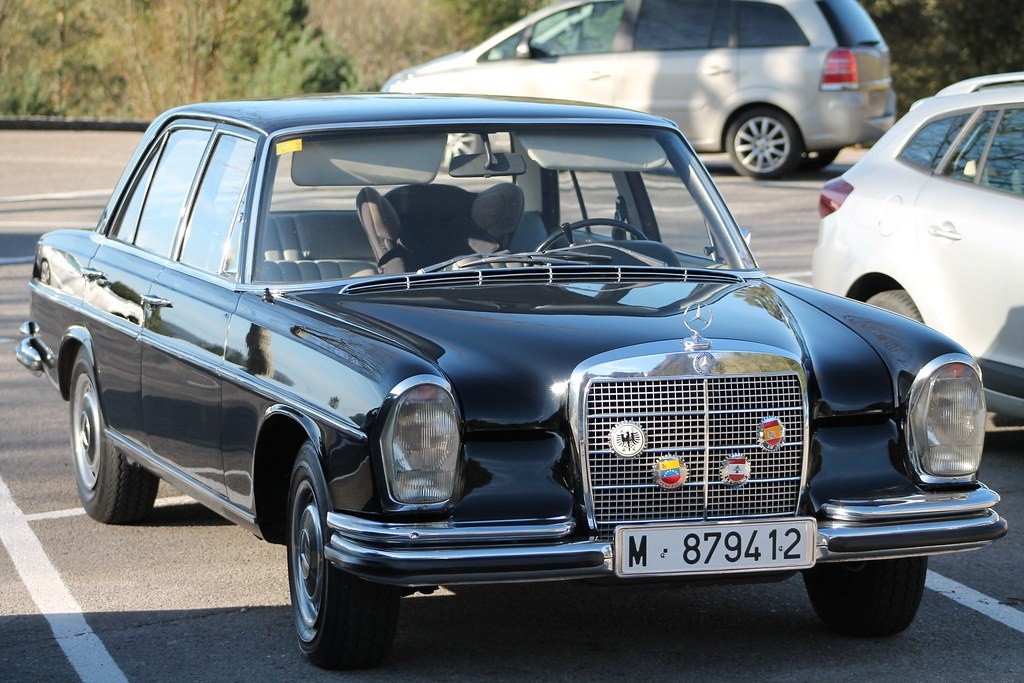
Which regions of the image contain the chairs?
[256,259,376,282]
[357,183,524,274]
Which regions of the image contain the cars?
[12,89,1011,671]
[374,0,896,183]
[810,72,1024,420]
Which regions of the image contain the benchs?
[260,208,547,265]
[977,145,1024,194]
